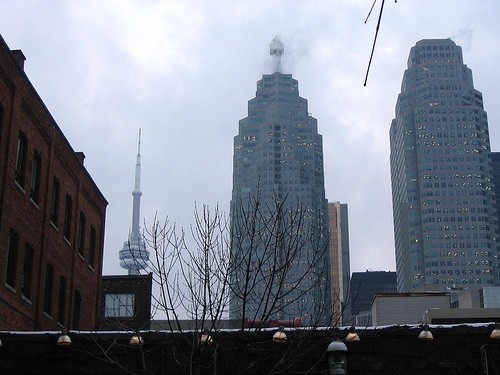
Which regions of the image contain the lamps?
[271,326,286,342]
[130,328,144,345]
[418,323,433,340]
[489,322,500,339]
[56,329,71,346]
[199,327,212,344]
[346,326,360,342]
